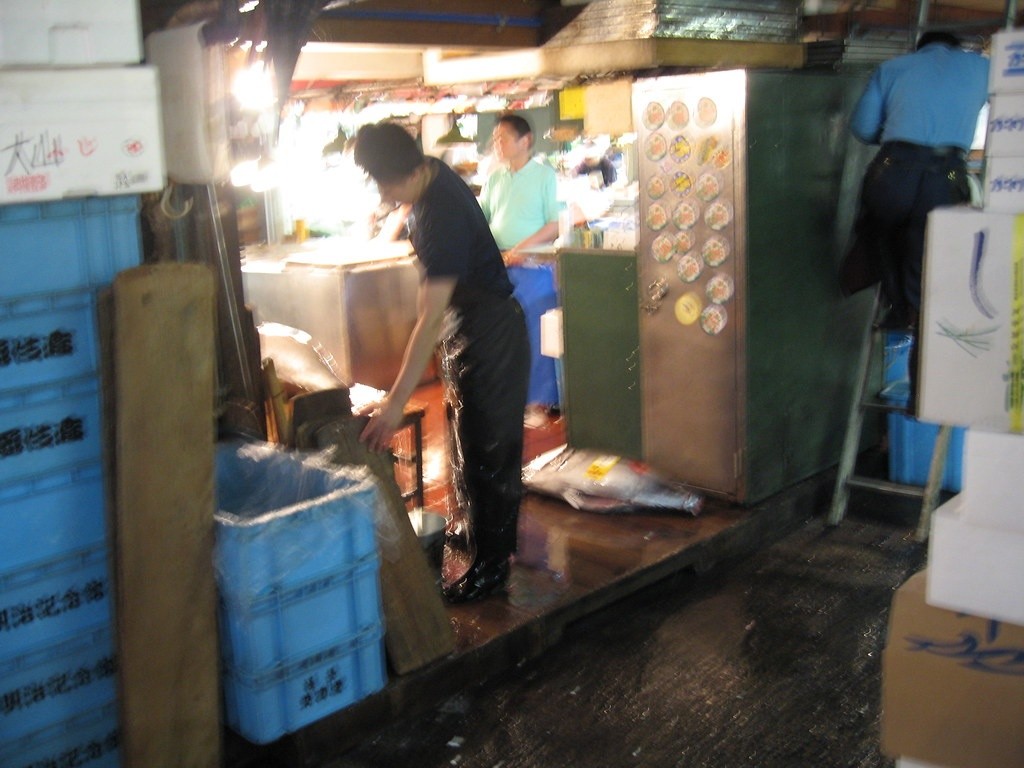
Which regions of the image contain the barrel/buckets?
[408,512,448,593]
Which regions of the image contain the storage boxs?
[0,62,389,768]
[878,28,1022,767]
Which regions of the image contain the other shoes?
[445,560,512,604]
[445,531,468,551]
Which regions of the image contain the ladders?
[824,0,1023,531]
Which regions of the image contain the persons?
[846,32,993,416]
[569,140,617,245]
[472,114,558,416]
[354,123,534,604]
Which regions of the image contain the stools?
[356,394,426,510]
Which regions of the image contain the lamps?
[437,109,477,149]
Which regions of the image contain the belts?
[884,140,963,159]
